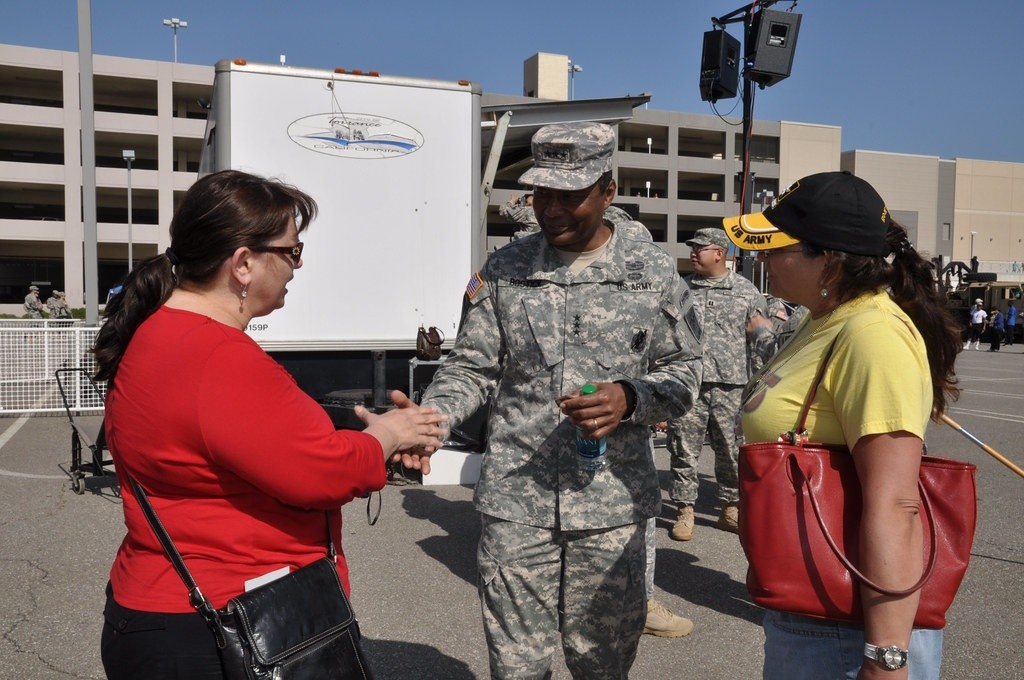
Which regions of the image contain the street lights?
[123,149,135,273]
[163,18,188,62]
[970,232,977,264]
[755,190,774,294]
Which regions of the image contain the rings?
[593,418,598,431]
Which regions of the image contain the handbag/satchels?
[417,327,444,360]
[57,307,74,327]
[209,557,373,680]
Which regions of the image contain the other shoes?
[987,349,999,352]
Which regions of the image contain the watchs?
[864,642,909,670]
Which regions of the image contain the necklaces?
[734,303,844,445]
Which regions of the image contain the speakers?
[743,9,802,87]
[700,30,741,101]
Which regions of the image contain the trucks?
[198,60,651,420]
[945,273,1024,338]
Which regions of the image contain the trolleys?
[55,366,114,495]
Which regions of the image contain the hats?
[686,228,729,249]
[722,171,895,265]
[522,189,534,197]
[975,298,983,306]
[29,286,39,290]
[518,121,615,191]
[52,290,65,296]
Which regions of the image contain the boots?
[974,341,979,351]
[963,340,971,350]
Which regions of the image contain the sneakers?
[671,506,694,541]
[738,313,976,629]
[718,506,738,534]
[643,598,694,638]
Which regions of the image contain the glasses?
[757,249,803,262]
[257,242,303,263]
[692,247,719,253]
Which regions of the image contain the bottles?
[574,385,607,470]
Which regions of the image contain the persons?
[746,291,809,373]
[963,298,1017,352]
[91,170,448,680]
[667,227,767,542]
[25,286,43,320]
[391,121,703,680]
[500,191,541,239]
[603,205,693,638]
[724,172,964,680]
[47,290,73,328]
